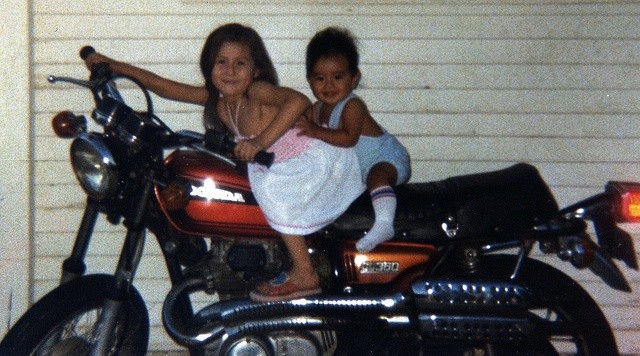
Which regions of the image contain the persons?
[291,26,412,253]
[85,24,367,301]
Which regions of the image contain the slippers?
[249,271,323,303]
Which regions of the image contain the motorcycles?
[0,46,640,355]
